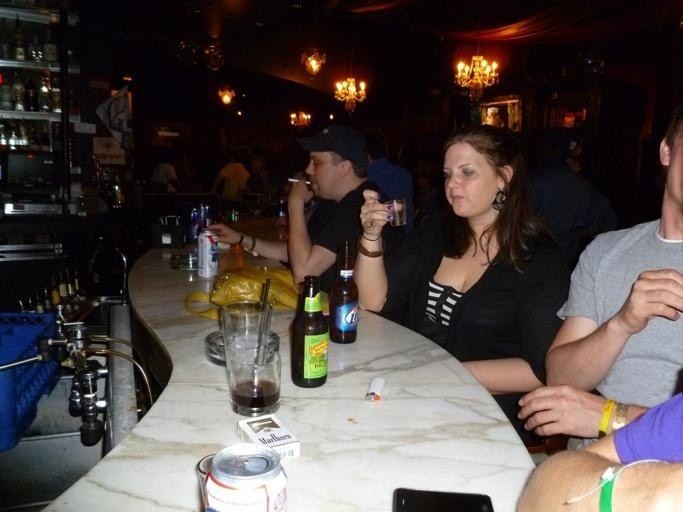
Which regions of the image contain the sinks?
[0,308,107,512]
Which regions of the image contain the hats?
[295,126,363,162]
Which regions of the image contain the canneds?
[190,204,213,238]
[231,210,240,221]
[205,441,289,512]
[197,231,219,279]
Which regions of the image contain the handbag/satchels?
[211,266,297,316]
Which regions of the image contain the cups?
[229,350,281,417]
[196,454,216,511]
[217,301,273,387]
[384,199,408,227]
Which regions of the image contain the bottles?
[6,267,82,314]
[1,13,63,113]
[329,238,360,346]
[290,273,329,389]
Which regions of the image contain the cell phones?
[393,488,493,512]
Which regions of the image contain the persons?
[212,105,683,512]
[151,147,181,192]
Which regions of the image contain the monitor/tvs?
[1,150,65,204]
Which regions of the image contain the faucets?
[38,303,87,357]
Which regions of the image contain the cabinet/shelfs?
[0,0,96,262]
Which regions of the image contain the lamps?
[301,39,500,115]
